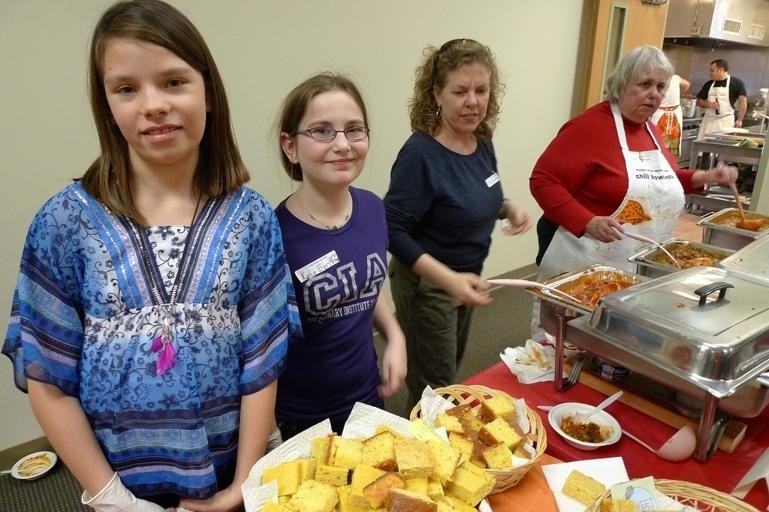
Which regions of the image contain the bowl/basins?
[11,451,57,480]
[547,403,622,450]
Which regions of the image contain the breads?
[431,394,532,472]
[257,420,497,511]
[561,469,637,512]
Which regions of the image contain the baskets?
[587,480,757,512]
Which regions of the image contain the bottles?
[716,98,720,114]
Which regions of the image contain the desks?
[477,454,570,511]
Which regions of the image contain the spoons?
[570,391,623,425]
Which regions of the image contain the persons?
[386,38,533,420]
[529,46,739,332]
[649,74,692,156]
[698,57,749,168]
[267,71,407,453]
[0,0,303,512]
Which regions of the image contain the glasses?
[294,126,369,141]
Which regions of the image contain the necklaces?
[119,186,205,375]
[290,193,353,233]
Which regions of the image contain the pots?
[680,96,698,118]
